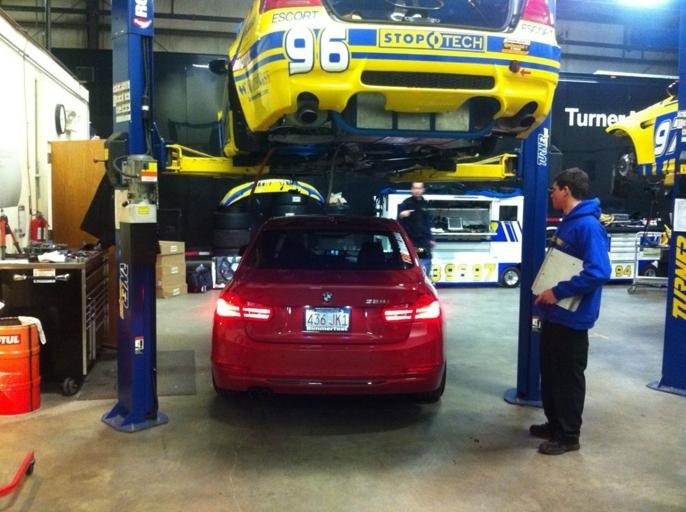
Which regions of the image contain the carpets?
[76,349,197,396]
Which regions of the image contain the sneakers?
[530,423,580,455]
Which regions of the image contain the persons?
[528,165,614,455]
[397,179,437,279]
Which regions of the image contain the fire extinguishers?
[31,211,49,243]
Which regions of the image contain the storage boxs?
[154,240,189,299]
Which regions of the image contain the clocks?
[56,104,66,134]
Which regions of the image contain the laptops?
[448,217,464,232]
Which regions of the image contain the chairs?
[272,235,386,270]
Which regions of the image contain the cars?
[605,80,685,179]
[209,214,447,402]
[208,1,561,171]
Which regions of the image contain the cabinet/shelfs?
[0,246,110,397]
